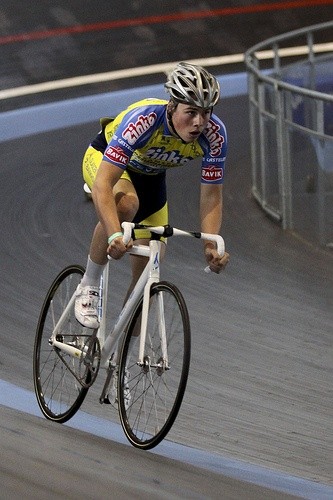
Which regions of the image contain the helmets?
[163,62,222,109]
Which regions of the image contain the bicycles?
[33,220,226,450]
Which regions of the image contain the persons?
[74,61,228,414]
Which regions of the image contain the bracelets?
[107,231,122,245]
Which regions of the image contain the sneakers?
[105,353,132,411]
[74,283,100,329]
